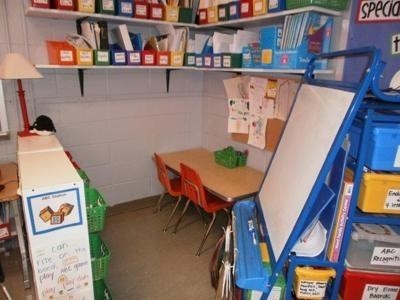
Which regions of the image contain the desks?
[152,148,265,202]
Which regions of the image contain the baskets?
[215,146,247,168]
[86,187,107,233]
[90,233,110,282]
[286,0,347,10]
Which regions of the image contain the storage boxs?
[290,267,336,300]
[341,271,400,300]
[343,167,400,215]
[341,222,400,274]
[343,121,400,171]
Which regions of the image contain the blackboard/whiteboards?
[256,79,363,274]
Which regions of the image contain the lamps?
[0,52,44,137]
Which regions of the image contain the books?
[66,0,332,52]
[328,169,355,262]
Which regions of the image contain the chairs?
[153,153,202,232]
[174,164,229,255]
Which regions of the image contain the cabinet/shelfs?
[24,7,342,97]
[286,109,400,300]
[18,129,95,300]
